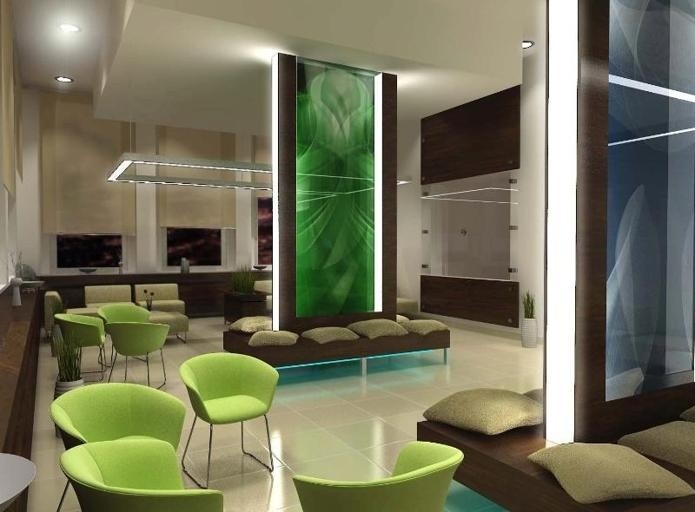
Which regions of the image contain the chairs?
[59,438,224,511]
[49,382,187,512]
[292,439,465,512]
[44,282,189,344]
[53,303,170,389]
[179,350,280,489]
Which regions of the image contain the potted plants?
[54,334,85,399]
[216,264,268,325]
[520,289,538,348]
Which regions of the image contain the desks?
[0,285,41,512]
[420,274,519,328]
[35,272,273,319]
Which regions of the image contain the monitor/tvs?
[167,229,222,266]
[56,234,123,268]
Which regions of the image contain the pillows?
[421,387,543,436]
[229,315,451,348]
[526,440,695,504]
[617,421,695,472]
[679,405,695,422]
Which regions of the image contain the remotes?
[253,265,266,269]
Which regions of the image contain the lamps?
[104,118,273,191]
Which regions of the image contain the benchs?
[417,420,695,512]
[222,313,450,376]
